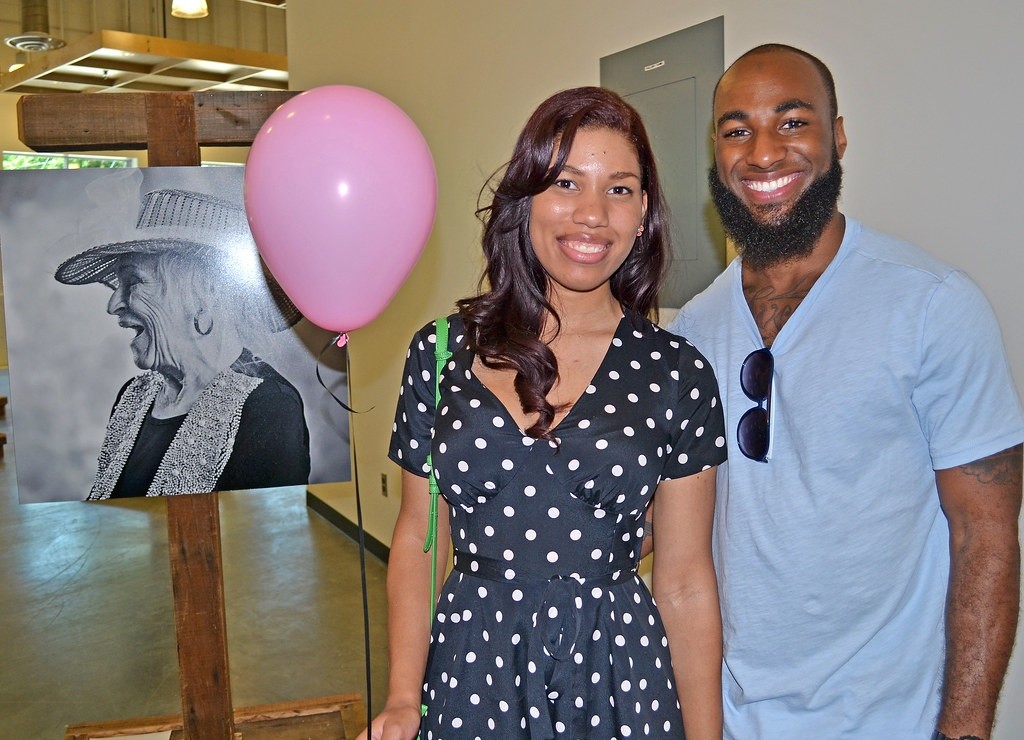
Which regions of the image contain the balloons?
[245,83,431,335]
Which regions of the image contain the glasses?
[735,344,774,465]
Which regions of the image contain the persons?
[663,43,1024,740]
[52,191,311,502]
[356,88,728,740]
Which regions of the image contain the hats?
[55,192,304,332]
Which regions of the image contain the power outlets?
[381,474,388,496]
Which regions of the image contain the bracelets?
[931,730,983,740]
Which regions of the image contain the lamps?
[170,0,208,19]
